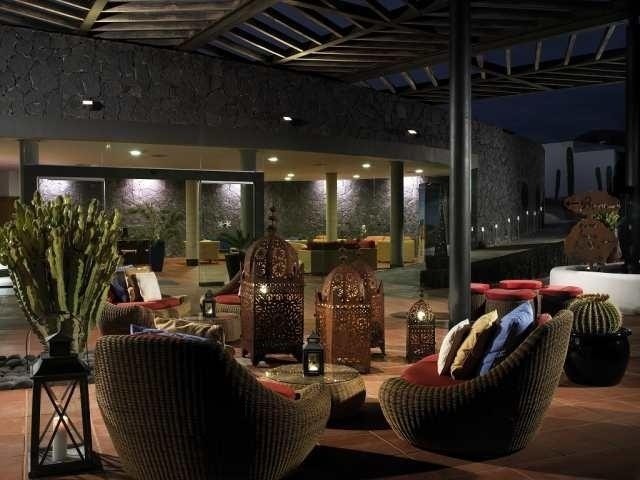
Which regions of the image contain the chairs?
[199,269,242,316]
[377,309,573,461]
[95,335,333,479]
[96,261,184,335]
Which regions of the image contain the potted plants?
[125,200,186,271]
[562,292,633,387]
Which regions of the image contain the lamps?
[202,289,215,318]
[302,334,324,376]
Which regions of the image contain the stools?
[470,279,583,322]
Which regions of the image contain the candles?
[52,416,68,462]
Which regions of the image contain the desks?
[265,363,366,422]
[181,312,241,343]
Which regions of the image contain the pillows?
[437,300,553,381]
[111,264,163,303]
[130,314,236,356]
